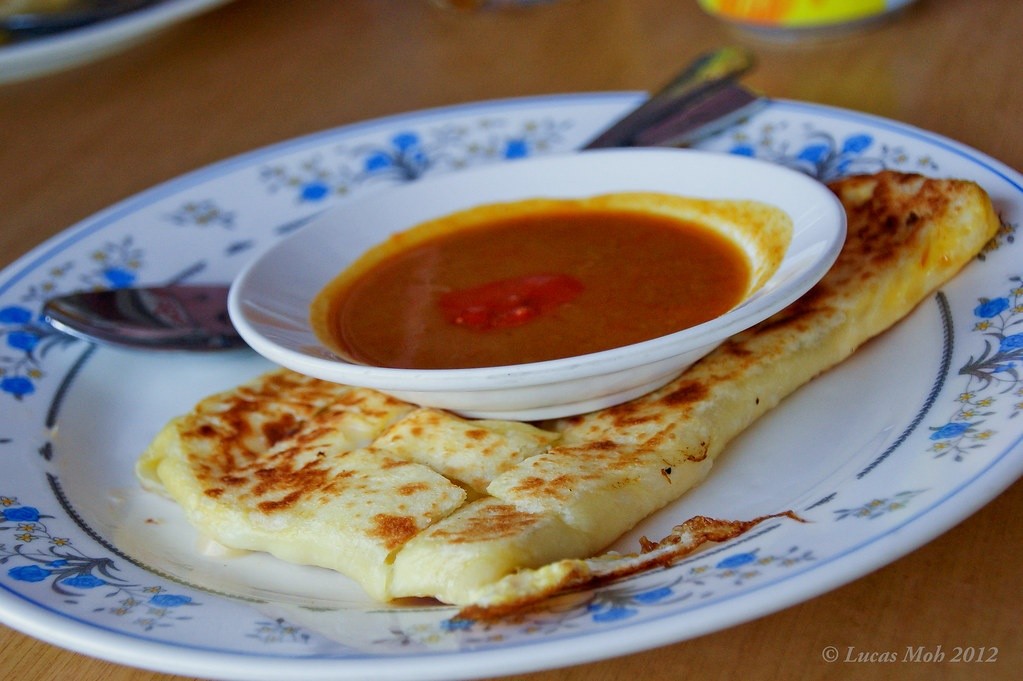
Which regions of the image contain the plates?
[229,146,847,423]
[0,0,230,83]
[0,93,1023,681]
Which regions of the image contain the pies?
[131,154,1003,616]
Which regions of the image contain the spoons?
[42,43,758,351]
